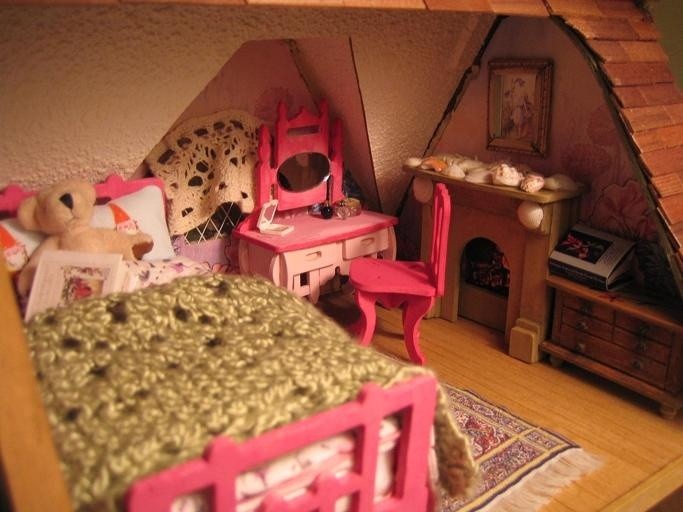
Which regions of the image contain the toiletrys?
[336,201,350,219]
[320,200,334,217]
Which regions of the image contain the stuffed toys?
[11,182,156,298]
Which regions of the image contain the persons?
[508,78,529,142]
